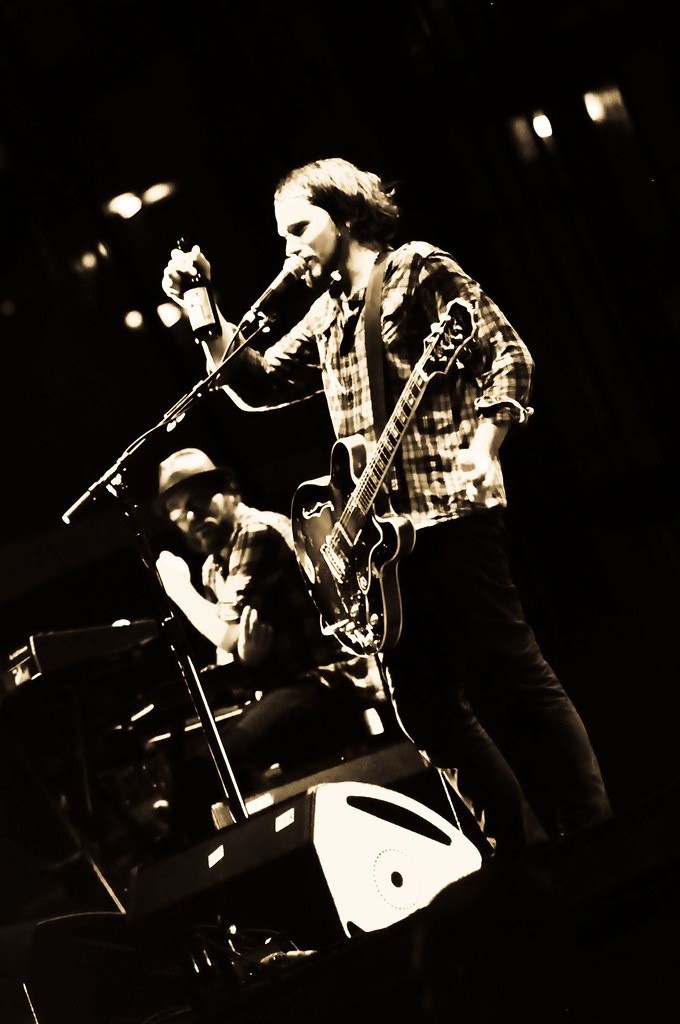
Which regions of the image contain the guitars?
[285,295,484,660]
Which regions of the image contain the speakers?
[127,779,483,1004]
[0,912,176,1024]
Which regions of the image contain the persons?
[154,447,349,833]
[162,158,615,853]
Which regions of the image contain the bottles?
[177,239,223,343]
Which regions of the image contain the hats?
[152,446,225,520]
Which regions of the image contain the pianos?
[0,616,160,704]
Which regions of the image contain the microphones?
[238,256,307,331]
